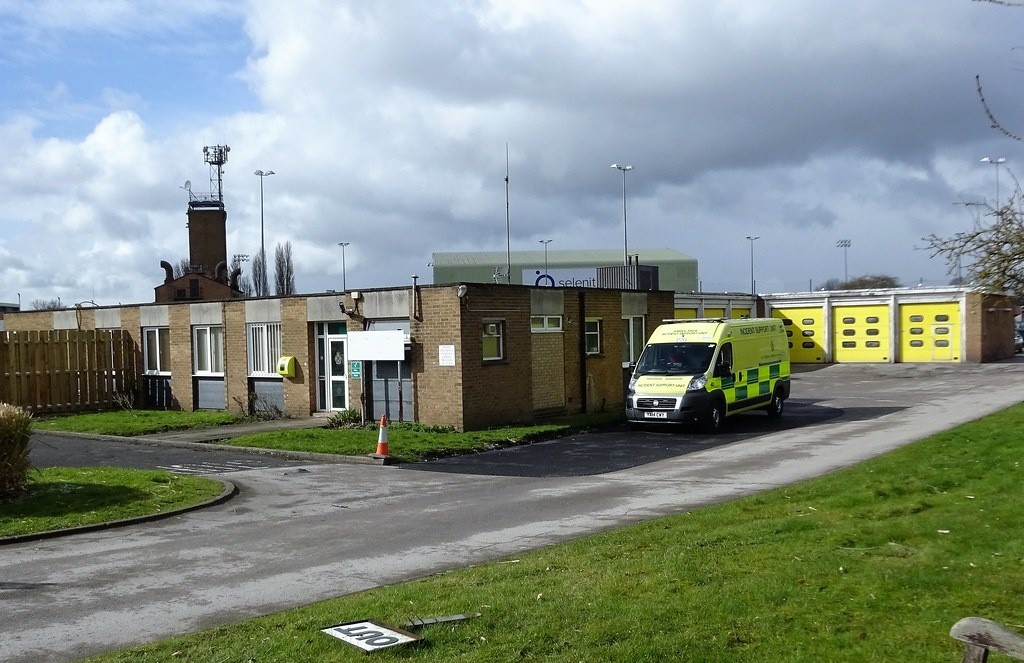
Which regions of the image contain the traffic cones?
[367,415,392,459]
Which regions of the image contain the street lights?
[539,240,554,287]
[609,162,636,266]
[979,155,1007,225]
[746,236,760,296]
[835,238,853,284]
[338,242,351,292]
[251,171,276,267]
[225,255,250,292]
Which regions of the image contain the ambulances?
[624,315,790,435]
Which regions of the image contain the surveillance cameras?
[339,302,346,312]
